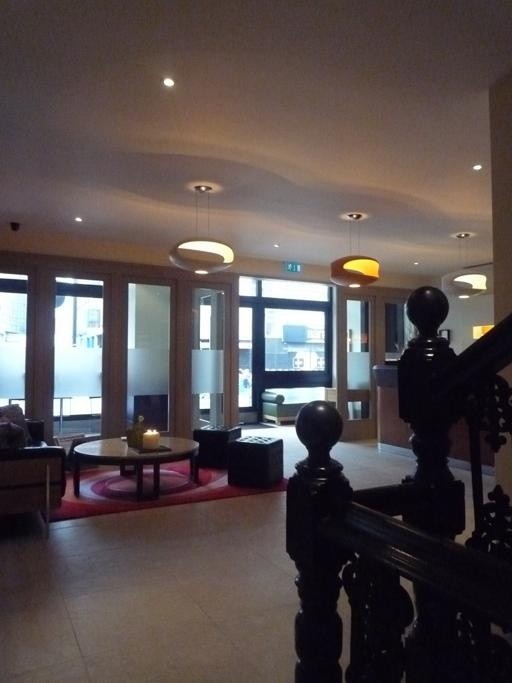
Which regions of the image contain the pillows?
[0,404,34,448]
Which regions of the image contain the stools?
[1,491,47,542]
[193,423,284,490]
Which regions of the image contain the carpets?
[46,466,289,523]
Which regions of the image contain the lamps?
[168,182,235,275]
[441,233,488,301]
[327,212,381,289]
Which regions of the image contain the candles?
[143,429,160,451]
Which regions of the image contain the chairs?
[0,403,68,511]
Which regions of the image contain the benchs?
[261,390,336,426]
